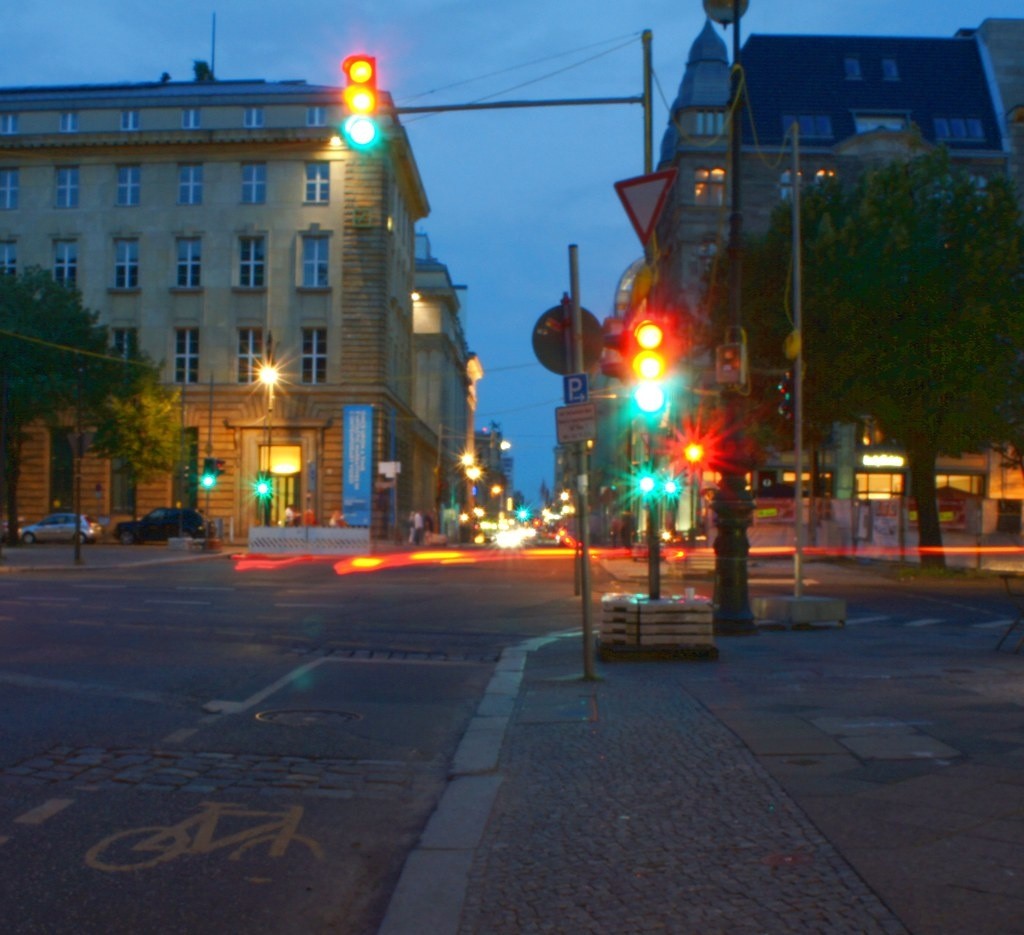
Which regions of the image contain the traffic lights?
[713,343,747,388]
[603,328,633,389]
[518,507,527,519]
[680,436,719,470]
[201,460,225,486]
[777,373,795,421]
[341,54,379,150]
[258,478,271,494]
[635,474,681,501]
[631,315,670,422]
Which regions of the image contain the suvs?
[112,508,216,543]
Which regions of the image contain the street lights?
[702,0,749,636]
[259,365,277,528]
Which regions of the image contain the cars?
[18,514,102,542]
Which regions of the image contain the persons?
[409,509,423,544]
[284,504,317,529]
[610,506,635,547]
[333,506,344,528]
